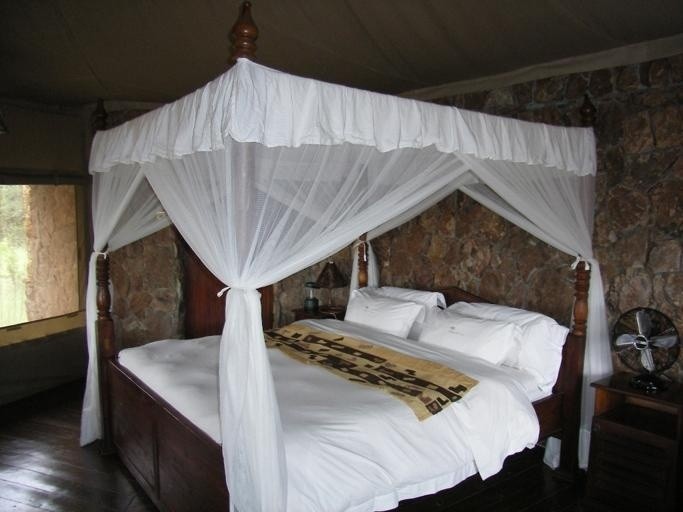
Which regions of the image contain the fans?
[611,306,681,391]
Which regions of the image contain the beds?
[75,0,609,512]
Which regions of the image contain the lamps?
[315,260,346,314]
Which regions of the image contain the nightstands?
[585,372,683,511]
[292,305,345,321]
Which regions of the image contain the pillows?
[342,289,426,340]
[443,300,562,354]
[370,285,445,339]
[419,304,519,368]
